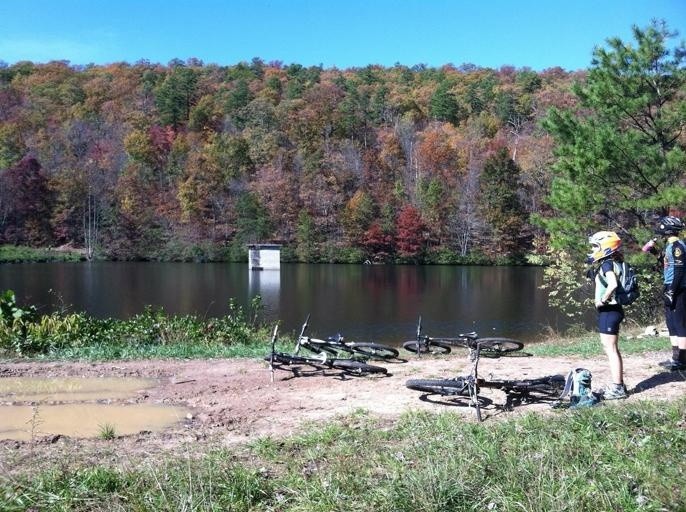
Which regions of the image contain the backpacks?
[552,368,597,409]
[594,258,640,305]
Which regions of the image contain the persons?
[588,230,628,400]
[641,214,685,374]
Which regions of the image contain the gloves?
[663,285,676,306]
[650,246,659,255]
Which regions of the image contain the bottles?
[641,237,659,253]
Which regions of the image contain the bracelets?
[601,298,606,306]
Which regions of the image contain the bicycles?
[404,316,524,358]
[295,315,399,363]
[406,344,566,423]
[263,325,388,384]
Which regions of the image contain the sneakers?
[603,383,628,400]
[659,358,686,371]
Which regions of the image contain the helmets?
[586,230,622,263]
[654,215,684,235]
